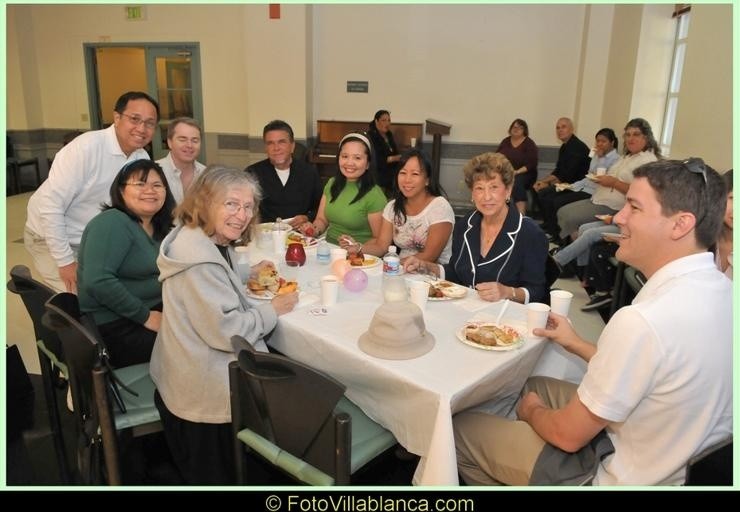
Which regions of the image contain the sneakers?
[582,293,613,311]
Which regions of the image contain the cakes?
[347,245,364,267]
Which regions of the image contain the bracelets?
[313,224,321,235]
[357,242,363,252]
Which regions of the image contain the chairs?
[6,265,163,486]
[229,351,398,486]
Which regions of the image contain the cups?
[278,261,300,279]
[523,301,551,338]
[411,138,416,148]
[319,277,340,309]
[596,167,607,177]
[549,290,574,320]
[231,220,318,268]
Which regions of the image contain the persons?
[77,158,176,367]
[147,162,297,485]
[403,114,732,485]
[299,130,388,250]
[367,110,404,190]
[337,146,457,267]
[155,113,207,223]
[245,120,320,231]
[24,87,160,294]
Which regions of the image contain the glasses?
[680,157,709,228]
[126,182,166,193]
[224,201,256,217]
[120,114,155,129]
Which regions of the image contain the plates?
[416,279,467,301]
[346,253,381,268]
[595,214,613,220]
[599,232,625,238]
[242,281,299,301]
[454,322,525,350]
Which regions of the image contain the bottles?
[383,276,409,305]
[382,244,400,296]
[315,240,331,277]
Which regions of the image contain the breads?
[466,324,513,345]
[248,281,265,294]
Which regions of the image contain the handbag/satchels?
[78,312,116,416]
